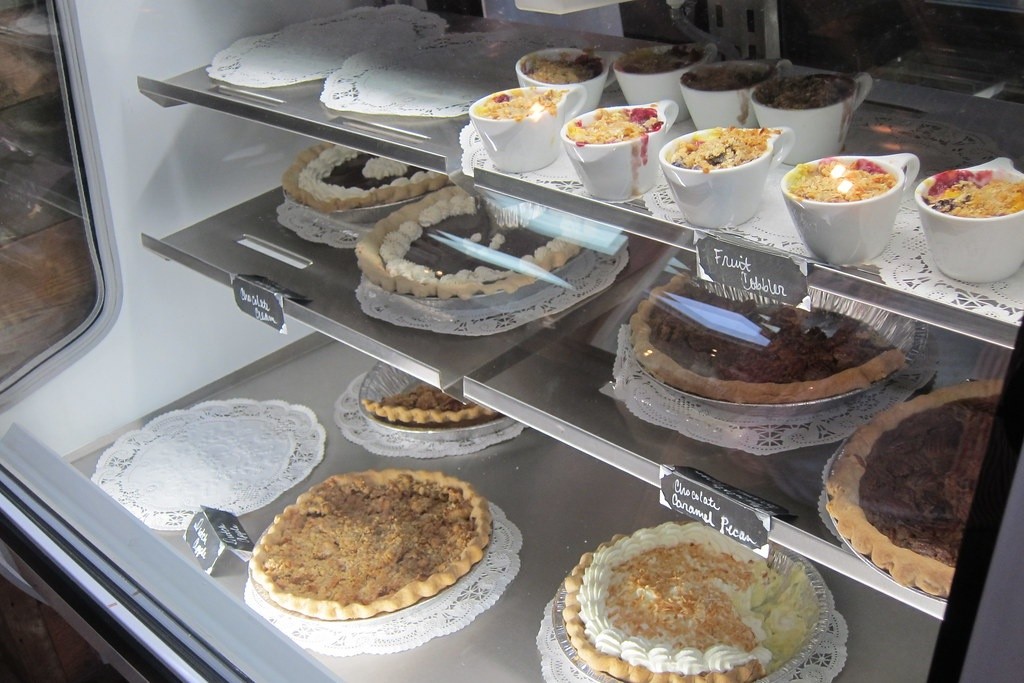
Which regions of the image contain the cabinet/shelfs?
[0,0,1023,681]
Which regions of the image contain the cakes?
[280,143,452,211]
[357,186,585,298]
[561,519,775,683]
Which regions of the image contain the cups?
[660,127,795,228]
[469,86,587,172]
[913,156,1024,282]
[750,72,872,167]
[613,42,718,123]
[560,99,680,200]
[780,152,921,265]
[515,46,624,117]
[679,57,793,130]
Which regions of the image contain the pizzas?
[825,376,1007,600]
[630,272,905,404]
[360,384,498,424]
[250,465,491,621]
